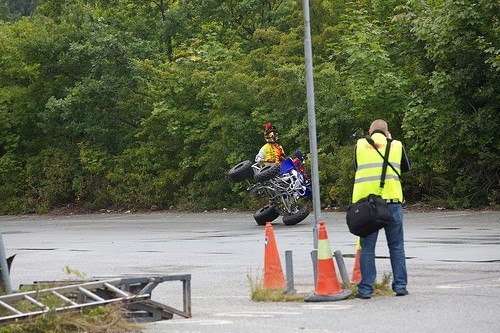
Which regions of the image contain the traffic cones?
[302,220,353,302]
[347,234,365,286]
[260,221,296,296]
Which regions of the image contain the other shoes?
[356,294,372,298]
[395,290,409,295]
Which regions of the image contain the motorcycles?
[228,150,313,226]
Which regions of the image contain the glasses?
[269,132,278,137]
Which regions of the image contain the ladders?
[0,277,150,324]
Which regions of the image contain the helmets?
[264,124,278,142]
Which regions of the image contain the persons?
[255,122,286,208]
[351,118,411,300]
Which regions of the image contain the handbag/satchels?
[345,195,393,237]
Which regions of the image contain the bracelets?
[267,159,269,162]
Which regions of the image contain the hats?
[295,151,304,162]
